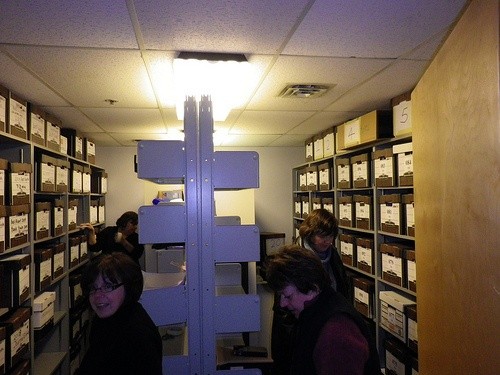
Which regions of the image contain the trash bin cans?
[260,231,286,281]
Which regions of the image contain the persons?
[271,209,349,363]
[79,211,144,258]
[72,252,164,375]
[266,245,382,375]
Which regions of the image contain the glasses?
[87,282,125,295]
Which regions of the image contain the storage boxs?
[293,95,419,375]
[0,83,108,375]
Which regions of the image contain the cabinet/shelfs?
[291,134,418,375]
[0,131,105,375]
[133,94,274,375]
